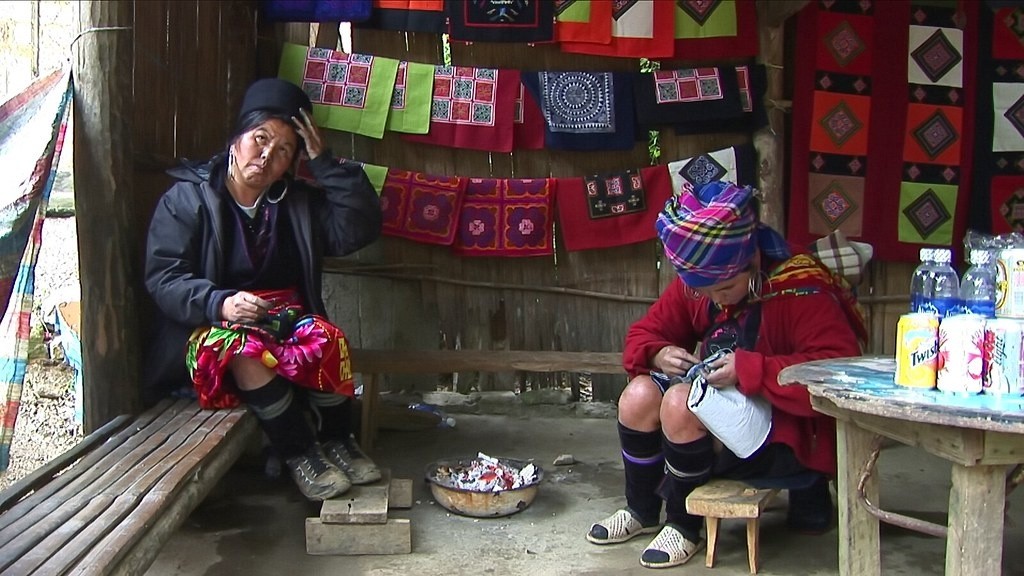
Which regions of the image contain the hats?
[236,79,313,148]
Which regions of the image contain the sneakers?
[285,442,351,502]
[322,434,382,484]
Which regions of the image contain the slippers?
[639,526,705,569]
[586,509,661,544]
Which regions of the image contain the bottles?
[960,249,996,319]
[407,402,456,427]
[922,249,959,321]
[911,250,936,313]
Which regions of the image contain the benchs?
[0,382,261,576]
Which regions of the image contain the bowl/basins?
[426,456,543,518]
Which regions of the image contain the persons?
[144,79,382,501]
[586,179,868,568]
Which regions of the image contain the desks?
[775,354,1024,576]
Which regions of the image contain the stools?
[683,477,783,575]
